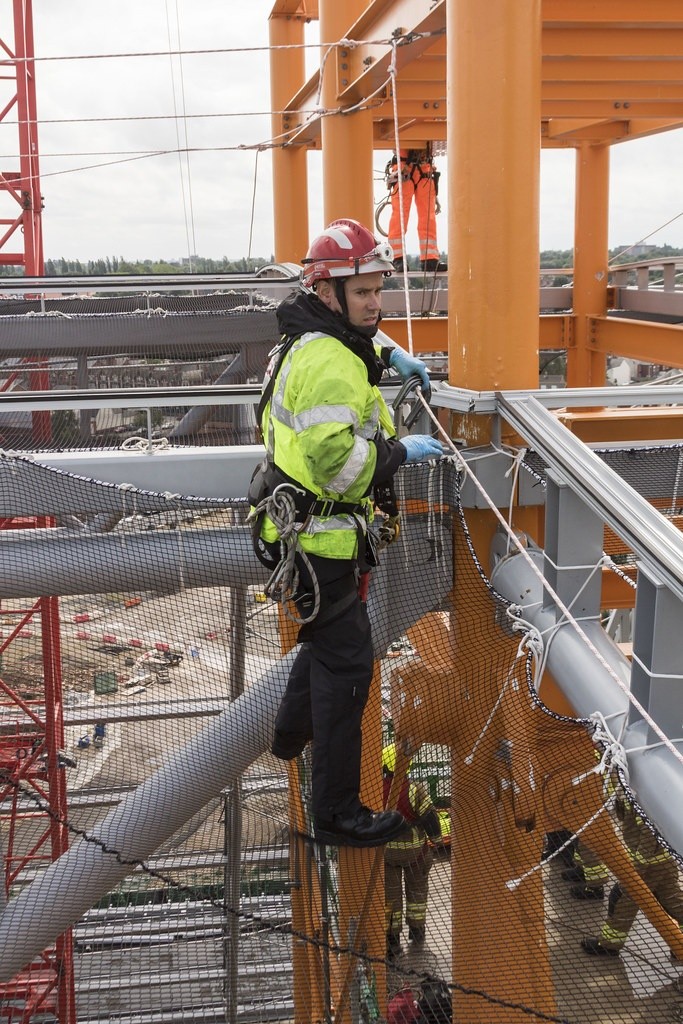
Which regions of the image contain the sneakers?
[313,806,409,849]
[270,729,313,760]
[392,257,410,273]
[420,259,448,271]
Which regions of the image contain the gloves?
[399,435,443,465]
[388,348,430,392]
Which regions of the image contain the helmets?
[302,219,396,287]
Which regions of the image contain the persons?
[384,150,449,273]
[380,743,449,963]
[247,217,446,850]
[543,789,682,954]
